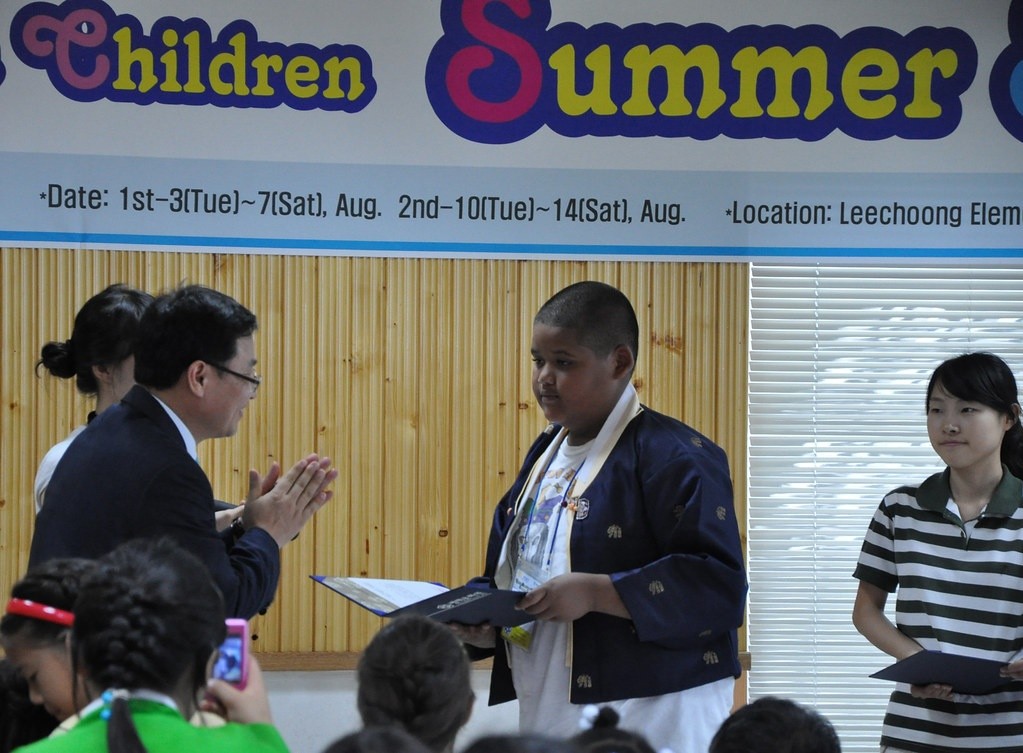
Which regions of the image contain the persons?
[25,284,338,621]
[464,732,572,753]
[357,616,476,753]
[443,281,749,753]
[0,553,227,727]
[851,349,1023,753]
[323,727,434,752]
[10,530,294,753]
[708,693,842,753]
[570,705,657,753]
[28,281,247,532]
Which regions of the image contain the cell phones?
[208,617,248,692]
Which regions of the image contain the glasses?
[184,358,262,393]
